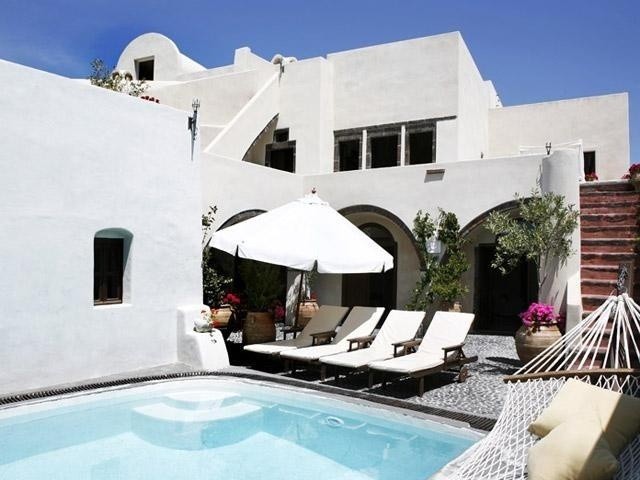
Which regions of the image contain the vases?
[212,304,235,338]
[515,321,565,372]
[294,300,319,326]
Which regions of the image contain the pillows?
[524,377,640,480]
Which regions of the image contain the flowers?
[519,301,563,326]
[223,293,241,305]
[621,163,640,179]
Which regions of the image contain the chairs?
[241,305,478,399]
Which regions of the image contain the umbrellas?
[209,187,394,337]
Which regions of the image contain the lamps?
[188,95,202,140]
